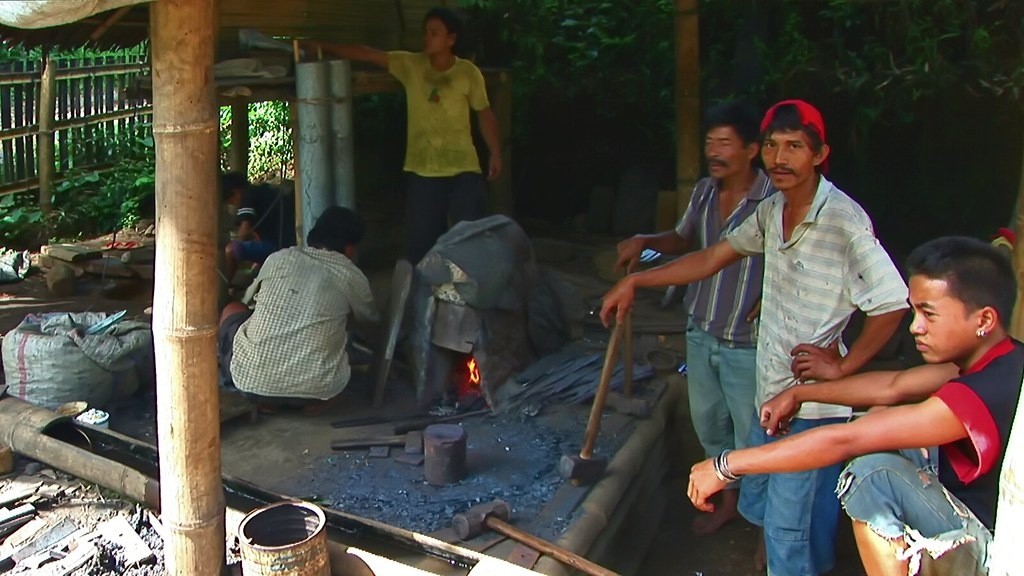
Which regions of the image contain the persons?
[224,172,282,285]
[216,207,379,418]
[289,9,502,265]
[599,100,912,576]
[613,108,772,572]
[687,236,1024,576]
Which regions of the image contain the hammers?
[606,263,652,417]
[558,313,626,486]
[453,497,623,576]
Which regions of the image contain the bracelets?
[712,449,746,483]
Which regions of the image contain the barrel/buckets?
[423,425,469,486]
[230,500,331,576]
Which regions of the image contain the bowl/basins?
[76,408,110,429]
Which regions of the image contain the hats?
[759,98,830,176]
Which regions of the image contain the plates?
[55,399,89,416]
